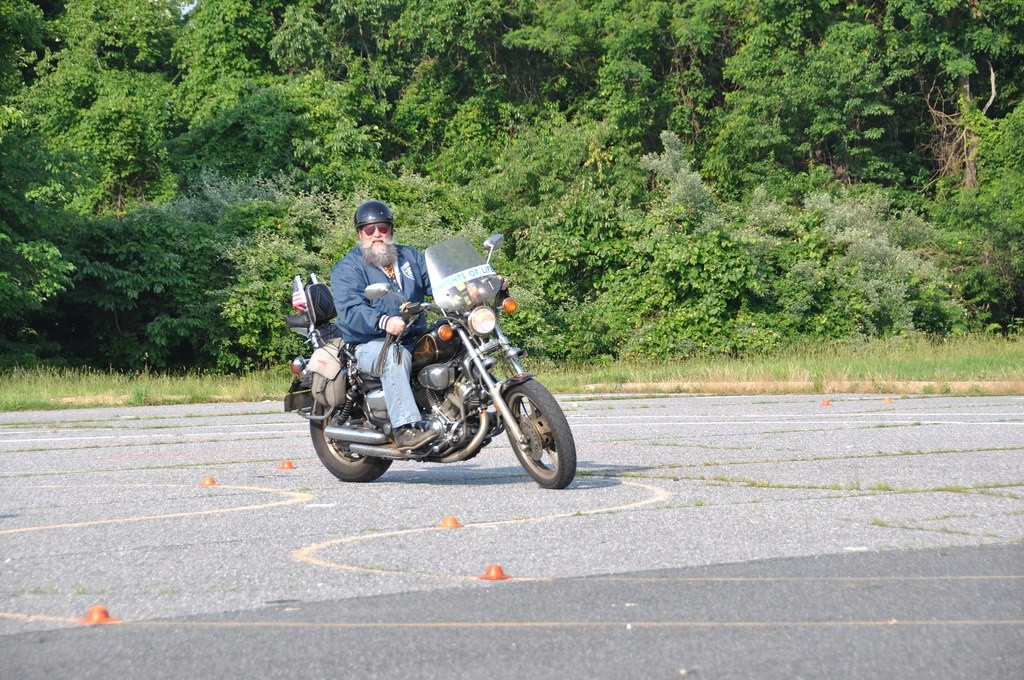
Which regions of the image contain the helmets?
[355,201,393,228]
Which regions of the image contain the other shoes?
[392,424,437,451]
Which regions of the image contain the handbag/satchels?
[307,338,347,407]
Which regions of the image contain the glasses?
[362,224,389,236]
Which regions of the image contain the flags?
[292,277,306,314]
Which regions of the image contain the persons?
[329,201,508,451]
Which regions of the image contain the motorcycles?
[279,234,578,490]
